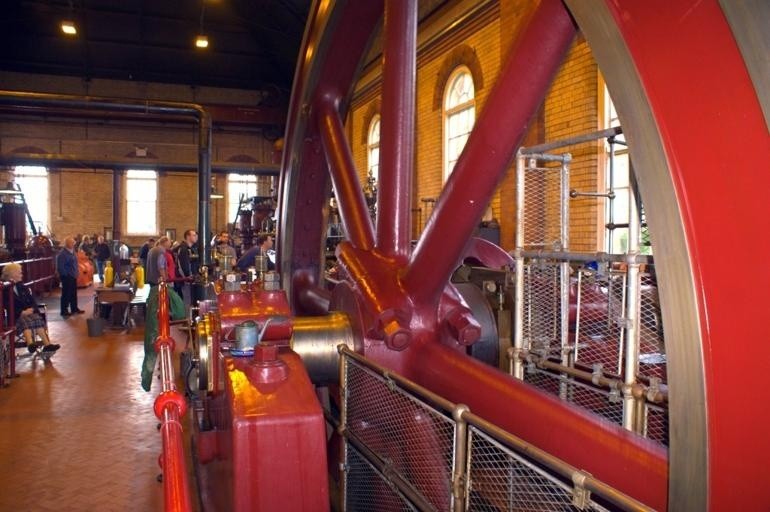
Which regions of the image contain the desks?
[94,280,131,336]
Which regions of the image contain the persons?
[0,260,61,354]
[55,226,276,320]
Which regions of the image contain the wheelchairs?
[4,280,56,362]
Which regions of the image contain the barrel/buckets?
[87,316,104,337]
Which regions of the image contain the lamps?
[61,20,80,35]
[194,36,209,48]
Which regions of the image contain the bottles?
[135,264,145,289]
[105,261,114,287]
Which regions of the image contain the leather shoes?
[60,309,85,316]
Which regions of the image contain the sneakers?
[28,344,60,353]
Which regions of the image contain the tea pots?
[225,318,273,351]
